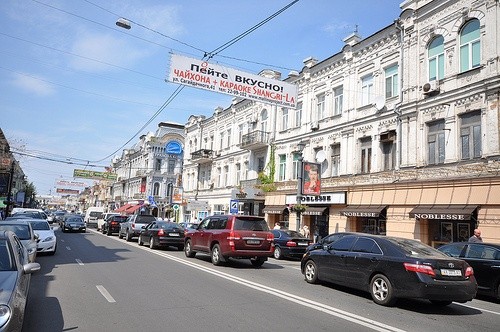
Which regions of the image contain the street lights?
[4,160,16,218]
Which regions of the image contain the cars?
[16,219,57,256]
[84,206,105,227]
[5,217,32,221]
[61,216,87,233]
[51,210,85,227]
[11,208,51,225]
[269,229,310,260]
[304,231,370,253]
[178,222,199,232]
[0,228,42,332]
[0,221,40,263]
[138,221,186,251]
[436,242,500,304]
[300,235,478,307]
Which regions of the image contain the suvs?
[95,212,121,232]
[182,215,276,268]
[101,215,129,236]
[118,214,158,242]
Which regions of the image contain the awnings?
[408,204,479,223]
[340,205,387,219]
[262,205,289,215]
[302,207,329,217]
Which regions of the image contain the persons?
[468,229,486,257]
[273,222,319,244]
[304,167,320,195]
